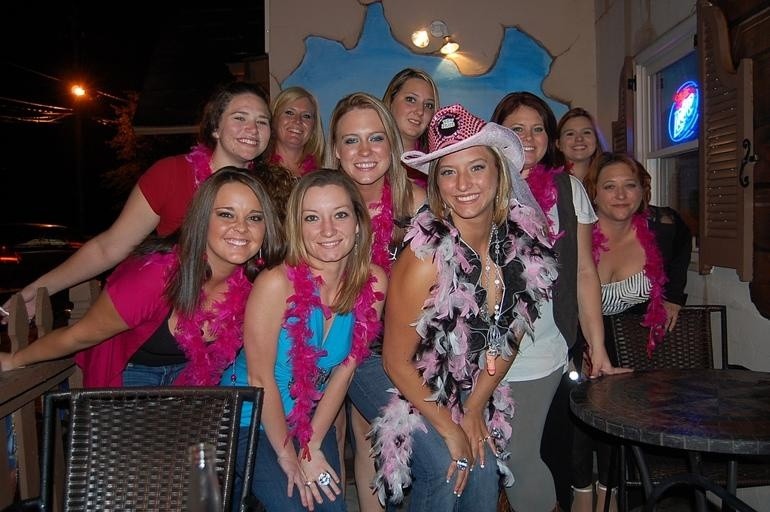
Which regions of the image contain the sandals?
[568,485,594,512]
[595,480,619,512]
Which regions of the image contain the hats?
[400,101,526,173]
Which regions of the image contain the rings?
[483,435,491,441]
[479,437,485,443]
[304,480,313,486]
[317,470,332,487]
[451,455,469,472]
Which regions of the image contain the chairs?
[635,467,760,510]
[611,297,750,512]
[1,380,266,512]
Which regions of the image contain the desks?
[565,367,769,507]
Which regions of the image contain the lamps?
[405,17,460,59]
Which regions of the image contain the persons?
[215,166,390,512]
[555,106,611,213]
[563,148,694,511]
[379,68,440,194]
[0,78,273,326]
[324,91,429,511]
[487,91,637,510]
[346,103,558,510]
[0,164,290,392]
[265,86,328,181]
